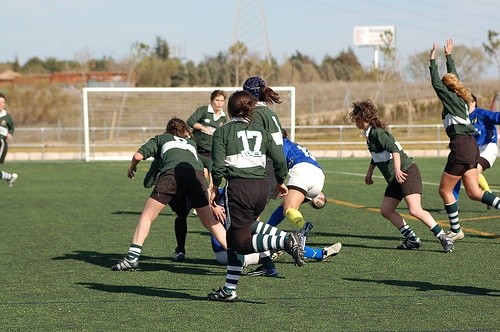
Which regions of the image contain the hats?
[243,76,266,99]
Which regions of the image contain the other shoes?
[439,208,447,213]
[487,191,495,210]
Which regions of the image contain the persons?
[144,152,214,262]
[208,90,309,302]
[439,93,500,214]
[0,93,19,188]
[242,77,314,278]
[213,185,343,267]
[350,101,456,254]
[186,89,229,217]
[429,38,500,242]
[110,117,227,272]
[267,129,326,242]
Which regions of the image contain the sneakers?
[446,229,465,240]
[298,232,306,251]
[111,256,139,271]
[247,266,278,277]
[318,241,342,262]
[173,253,186,262]
[208,286,238,301]
[299,222,314,236]
[288,232,305,266]
[396,237,423,250]
[440,235,456,252]
[8,173,18,188]
[270,248,283,261]
